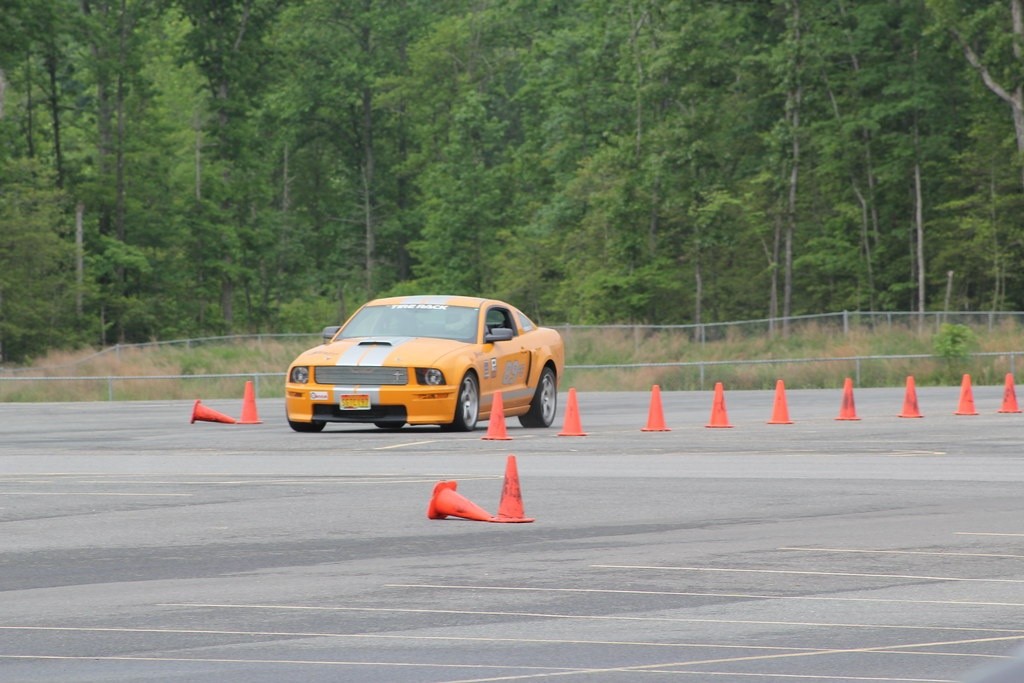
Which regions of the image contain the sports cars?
[283,292,566,435]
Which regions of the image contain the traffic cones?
[189,399,236,426]
[952,373,981,416]
[994,372,1023,414]
[558,386,587,438]
[481,390,514,442]
[897,376,924,419]
[704,380,734,429]
[837,378,862,421]
[491,456,537,526]
[767,379,794,425]
[427,481,498,522]
[642,385,674,433]
[236,380,262,425]
